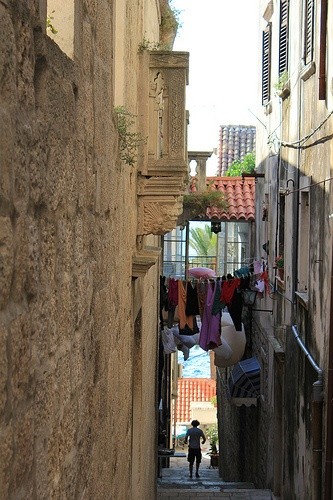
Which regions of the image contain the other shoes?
[189,475,192,477]
[196,475,200,477]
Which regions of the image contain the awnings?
[227,356,261,406]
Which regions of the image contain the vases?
[278,269,284,280]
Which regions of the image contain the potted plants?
[204,424,219,467]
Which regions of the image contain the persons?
[184,420,206,477]
[209,441,216,453]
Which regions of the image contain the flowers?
[273,256,284,268]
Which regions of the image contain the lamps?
[211,222,222,235]
